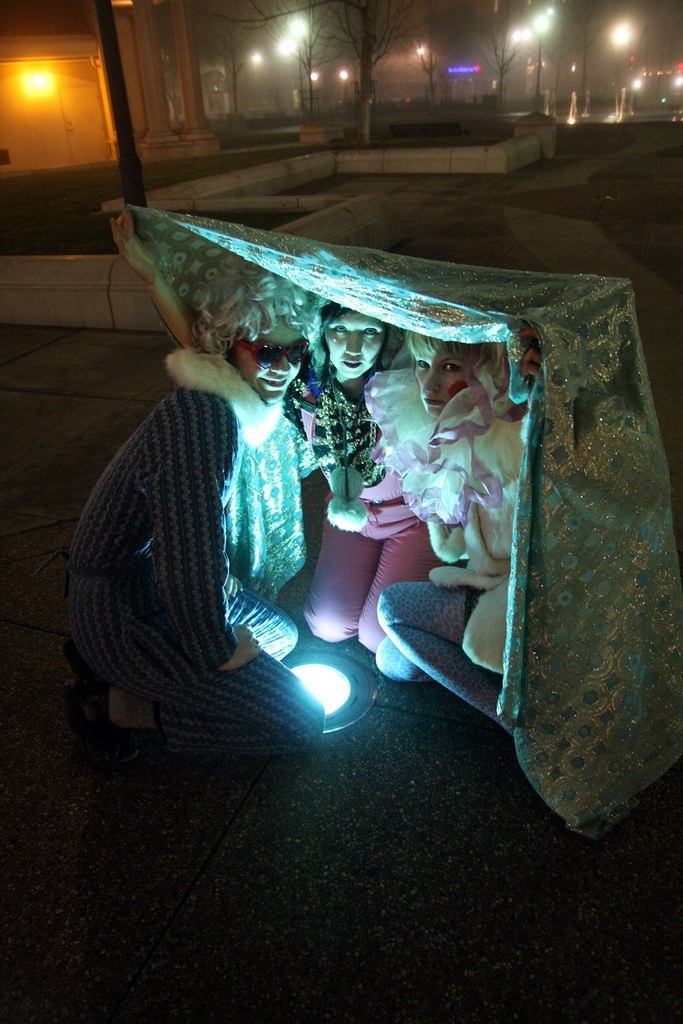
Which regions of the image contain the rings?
[254,639,259,646]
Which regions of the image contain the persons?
[111,210,443,654]
[363,329,542,738]
[34,265,325,757]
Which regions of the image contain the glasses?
[234,334,310,371]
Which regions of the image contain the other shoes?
[62,636,140,764]
